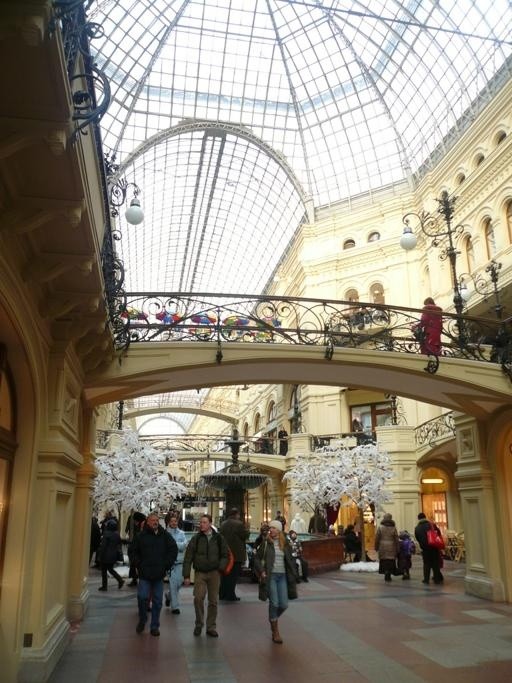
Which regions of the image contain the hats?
[268,519,284,534]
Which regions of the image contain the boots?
[270,619,283,643]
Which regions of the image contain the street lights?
[458,254,509,346]
[116,401,130,430]
[104,152,148,230]
[399,186,470,346]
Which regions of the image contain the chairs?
[443,530,465,562]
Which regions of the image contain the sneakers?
[98,578,138,592]
[171,607,180,615]
[385,569,442,585]
[164,591,171,608]
[207,629,218,637]
[195,625,202,635]
[136,621,145,632]
[149,627,160,636]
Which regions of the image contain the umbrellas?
[190,308,282,341]
[156,312,181,323]
[121,310,148,319]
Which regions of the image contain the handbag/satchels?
[215,532,233,574]
[252,539,269,583]
[426,521,444,548]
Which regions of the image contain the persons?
[411,297,442,355]
[375,513,398,581]
[278,427,288,455]
[415,513,445,584]
[399,531,416,580]
[432,549,444,580]
[90,510,371,644]
[352,416,363,431]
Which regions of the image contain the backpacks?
[403,538,415,555]
